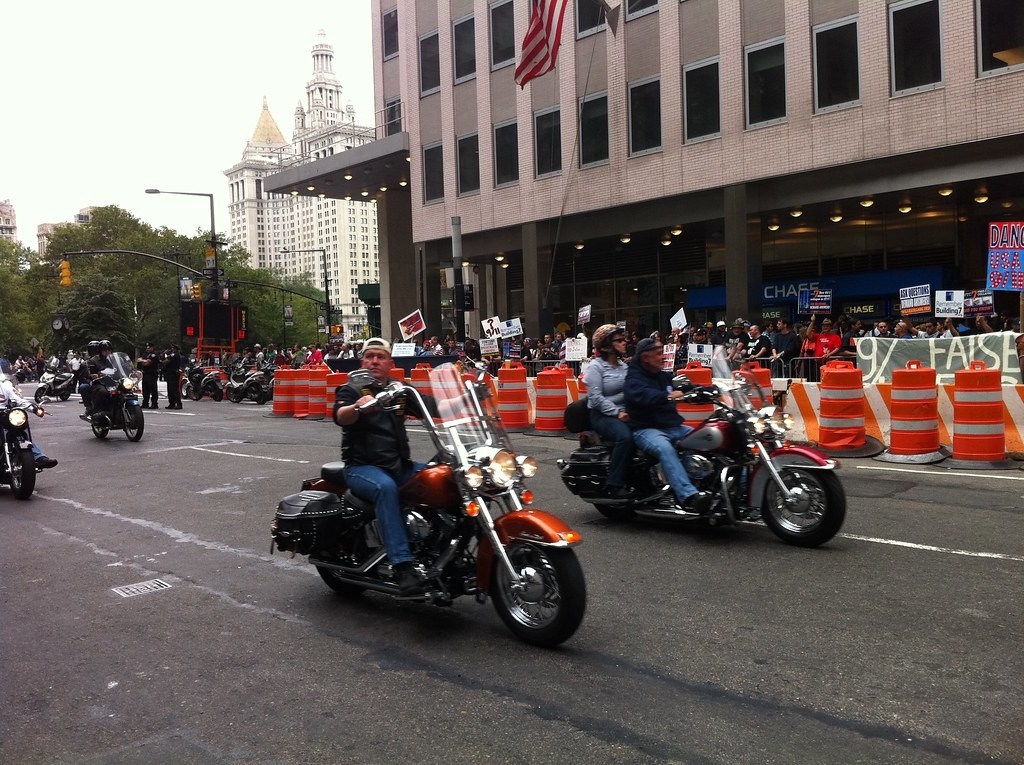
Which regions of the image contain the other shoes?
[79,408,91,418]
[164,405,175,409]
[34,456,60,469]
[175,405,182,410]
[688,493,711,510]
[148,405,158,409]
[603,483,633,497]
[140,403,149,409]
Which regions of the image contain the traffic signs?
[320,305,334,311]
[202,267,224,276]
[330,309,343,315]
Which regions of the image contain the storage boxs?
[274,490,341,554]
[561,448,612,496]
[564,398,594,432]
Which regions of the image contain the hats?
[423,340,430,344]
[254,344,261,349]
[630,338,664,362]
[360,337,391,353]
[146,342,153,346]
[820,318,832,327]
[171,343,181,351]
[697,318,751,334]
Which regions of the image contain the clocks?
[53,319,62,329]
[65,320,69,329]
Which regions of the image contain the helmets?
[87,339,99,353]
[98,339,114,352]
[589,323,625,348]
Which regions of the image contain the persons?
[0,375,58,470]
[410,330,589,377]
[623,339,720,509]
[581,323,632,498]
[331,338,473,590]
[76,339,141,423]
[137,342,188,409]
[204,340,363,372]
[0,352,84,394]
[669,314,1019,404]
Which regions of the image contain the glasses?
[879,326,887,328]
[609,338,626,343]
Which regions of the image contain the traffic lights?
[58,260,72,288]
[193,283,201,300]
[332,325,344,334]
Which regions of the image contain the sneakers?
[393,563,423,589]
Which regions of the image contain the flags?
[514,0,567,90]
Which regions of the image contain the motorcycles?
[267,362,590,650]
[13,361,47,384]
[554,343,848,549]
[33,357,77,404]
[178,356,282,405]
[78,351,145,443]
[0,371,53,500]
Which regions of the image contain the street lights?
[272,283,295,350]
[158,247,195,306]
[145,188,222,345]
[308,301,323,344]
[280,248,331,345]
